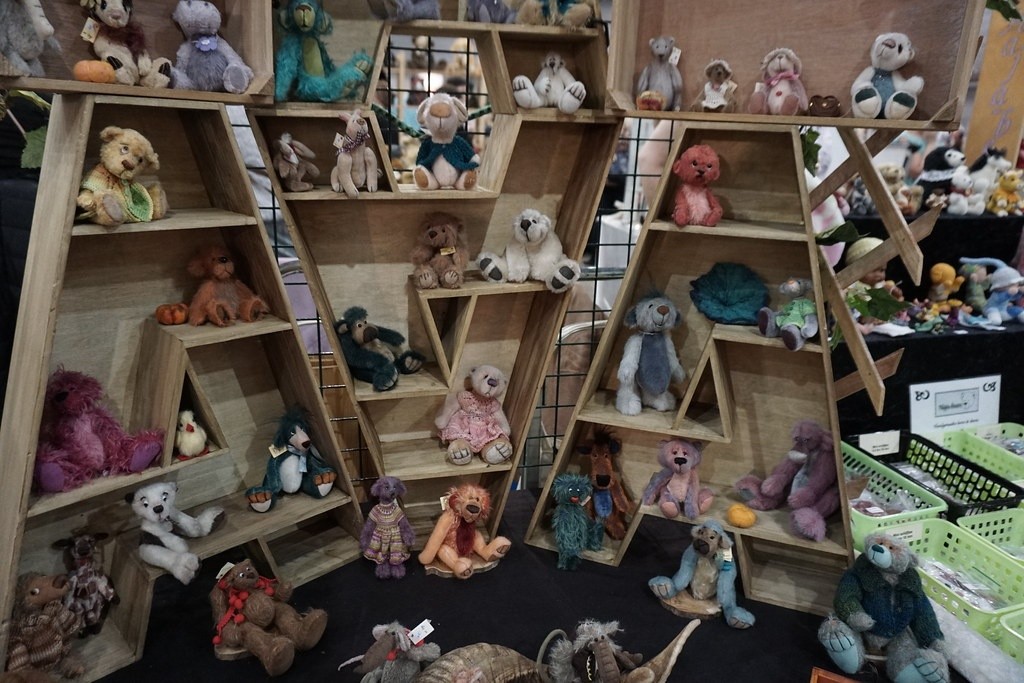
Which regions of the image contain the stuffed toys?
[838,237,1024,331]
[642,438,714,520]
[77,126,168,226]
[274,0,1024,219]
[352,618,702,683]
[187,241,270,327]
[359,475,415,580]
[732,419,840,543]
[175,410,209,460]
[334,207,580,393]
[0,0,255,94]
[33,363,166,493]
[615,276,818,415]
[244,402,337,513]
[434,365,513,465]
[208,557,328,675]
[418,481,512,579]
[549,421,638,571]
[0,571,91,683]
[648,520,756,628]
[51,532,121,639]
[124,480,225,585]
[817,531,952,683]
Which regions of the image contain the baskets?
[839,438,950,555]
[872,519,1024,640]
[849,431,1024,522]
[906,432,1024,505]
[962,421,1024,460]
[994,610,1024,665]
[957,508,1024,564]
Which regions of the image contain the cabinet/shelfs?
[0,0,1023,683]
